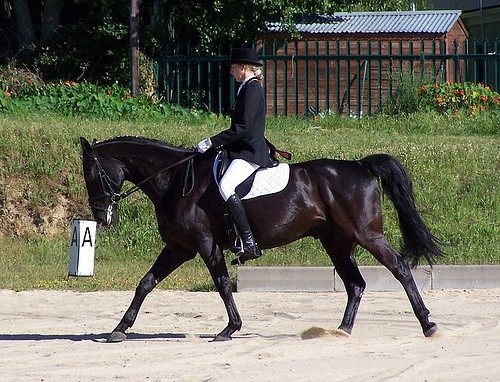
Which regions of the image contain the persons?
[194,43,271,265]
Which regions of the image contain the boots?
[225,194,261,265]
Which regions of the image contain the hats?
[228,48,264,67]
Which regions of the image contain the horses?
[79,136,451,343]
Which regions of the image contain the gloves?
[197,137,212,154]
